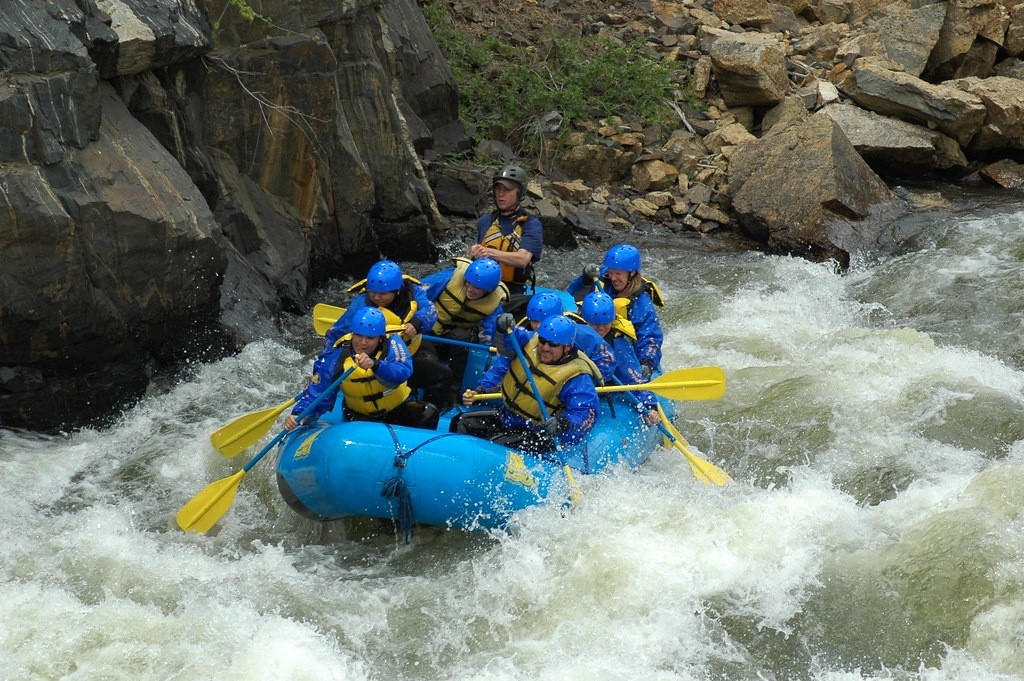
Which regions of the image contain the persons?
[579,292,660,427]
[418,258,503,345]
[570,244,664,379]
[470,165,543,294]
[461,294,618,409]
[448,312,598,452]
[284,307,439,430]
[322,260,450,403]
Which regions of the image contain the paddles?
[211,323,409,460]
[467,367,727,407]
[590,267,689,449]
[610,372,732,485]
[312,303,499,352]
[506,325,582,514]
[174,352,366,536]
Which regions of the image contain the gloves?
[642,364,653,379]
[542,416,561,436]
[584,263,601,279]
[498,313,514,331]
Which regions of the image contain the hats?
[492,178,521,190]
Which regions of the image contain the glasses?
[538,334,561,347]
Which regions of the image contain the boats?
[276,282,678,545]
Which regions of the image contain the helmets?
[603,243,642,274]
[464,257,502,293]
[492,164,527,199]
[526,290,562,321]
[582,291,615,325]
[537,315,578,347]
[349,306,387,336]
[366,261,403,293]
